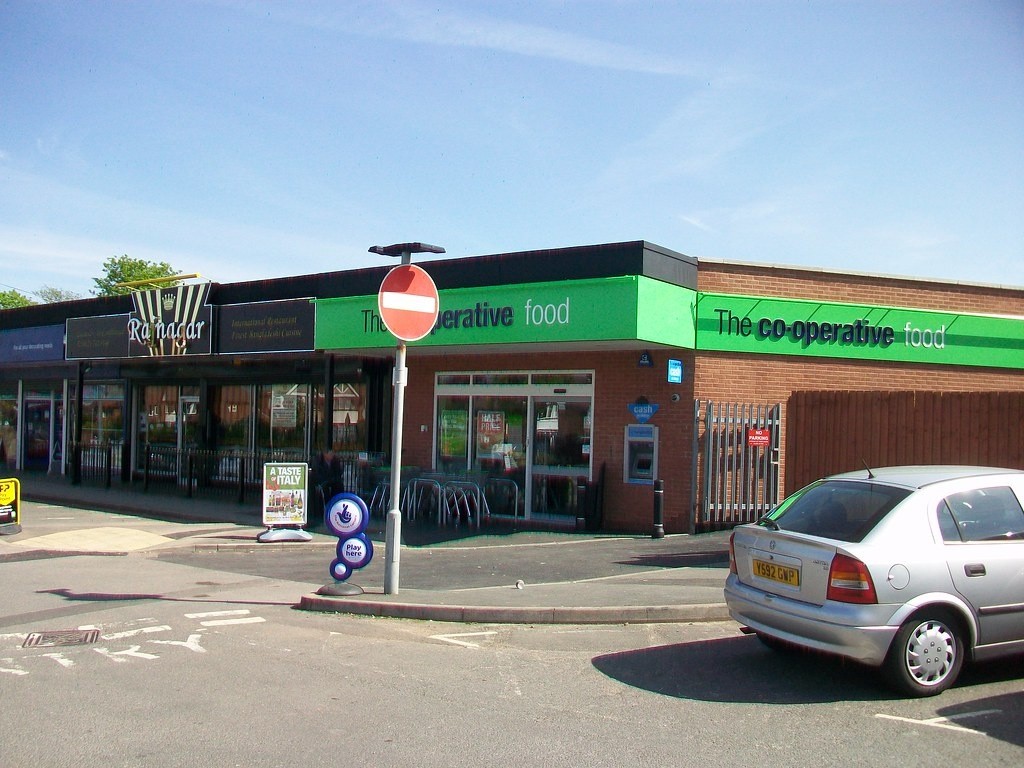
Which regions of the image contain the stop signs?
[377,264,440,342]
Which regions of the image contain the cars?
[724,464,1023,699]
